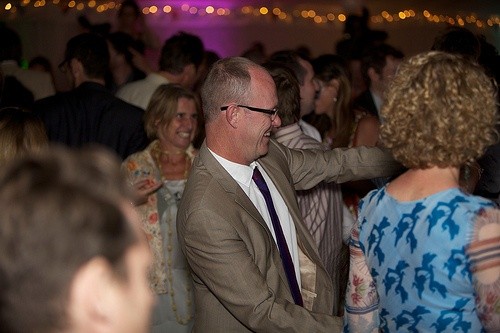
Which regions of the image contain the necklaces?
[154,143,196,325]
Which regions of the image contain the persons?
[258,62,344,314]
[24,31,151,166]
[120,82,199,333]
[176,56,408,333]
[0,0,266,164]
[0,141,156,333]
[342,49,500,333]
[267,13,500,211]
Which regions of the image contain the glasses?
[219,103,282,122]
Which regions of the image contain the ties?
[251,165,306,308]
